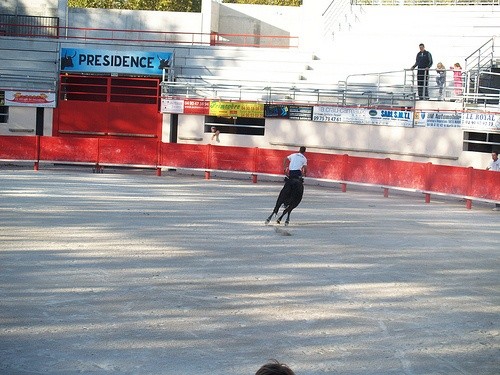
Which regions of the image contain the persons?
[484,151,500,213]
[208,126,221,140]
[450,63,464,95]
[410,44,433,100]
[435,63,447,95]
[283,146,308,179]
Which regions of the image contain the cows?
[265,172,304,226]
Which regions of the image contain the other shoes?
[492,208,500,210]
[281,205,286,208]
